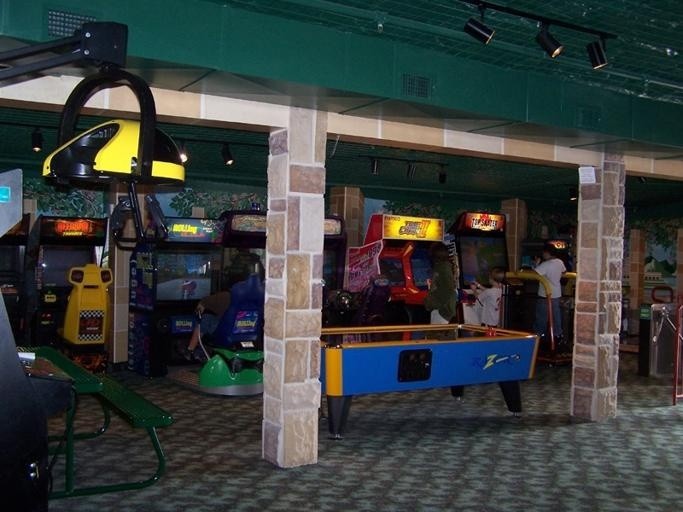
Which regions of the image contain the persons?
[531,243,566,344]
[172,274,244,361]
[471,268,504,327]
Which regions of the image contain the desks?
[14,348,104,499]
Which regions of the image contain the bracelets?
[476,283,481,288]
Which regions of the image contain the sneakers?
[176,341,194,359]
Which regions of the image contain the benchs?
[73,371,174,498]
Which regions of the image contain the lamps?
[462,0,620,70]
[31,127,43,153]
[177,138,234,166]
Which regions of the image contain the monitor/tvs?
[152,248,217,305]
[457,235,510,290]
[379,257,406,288]
[38,244,95,289]
[220,244,266,292]
[410,257,434,290]
